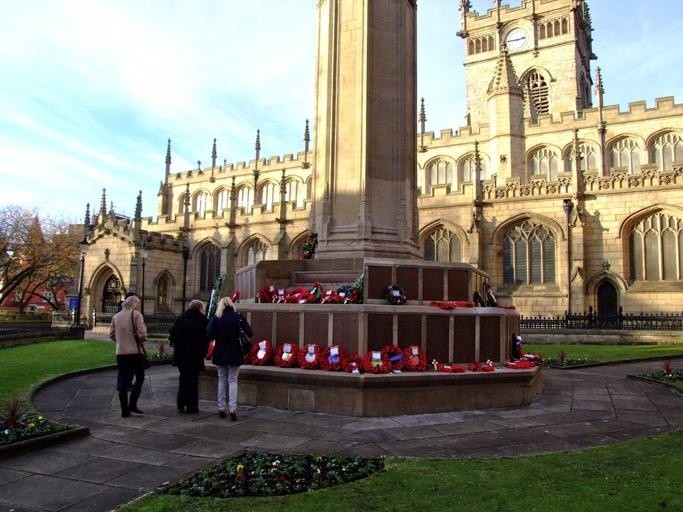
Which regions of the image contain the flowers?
[206,334,549,376]
[229,280,518,312]
[298,232,320,256]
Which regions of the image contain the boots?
[128,389,144,414]
[119,392,130,417]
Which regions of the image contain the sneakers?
[229,412,237,421]
[218,410,225,418]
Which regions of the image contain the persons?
[172,299,209,414]
[207,297,253,421]
[117,292,137,391]
[110,296,148,417]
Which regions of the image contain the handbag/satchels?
[239,330,251,353]
[206,339,215,360]
[138,354,152,369]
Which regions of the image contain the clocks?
[503,26,529,52]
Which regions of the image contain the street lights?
[136,241,152,315]
[71,235,90,340]
